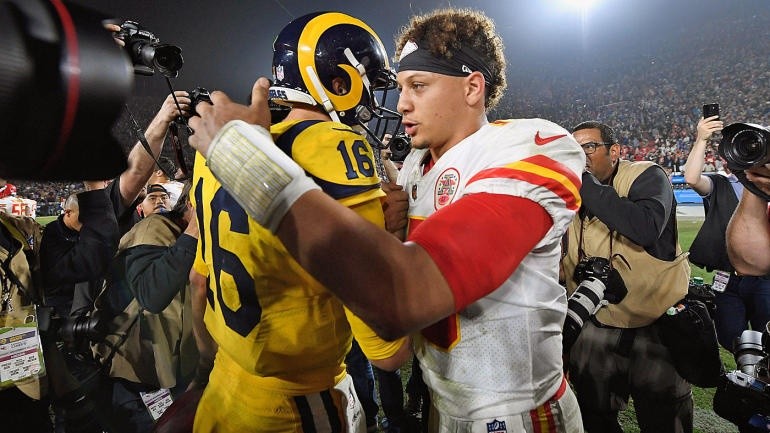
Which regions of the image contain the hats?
[147,184,168,195]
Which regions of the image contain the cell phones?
[703,103,719,121]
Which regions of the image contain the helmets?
[271,11,394,125]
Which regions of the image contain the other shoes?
[391,401,430,433]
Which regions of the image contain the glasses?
[580,141,616,155]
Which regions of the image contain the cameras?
[113,20,184,77]
[0,0,135,182]
[562,257,628,336]
[175,86,214,124]
[717,123,770,174]
[36,306,107,344]
[387,132,412,161]
[712,330,770,428]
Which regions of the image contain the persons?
[559,121,693,433]
[0,0,770,433]
